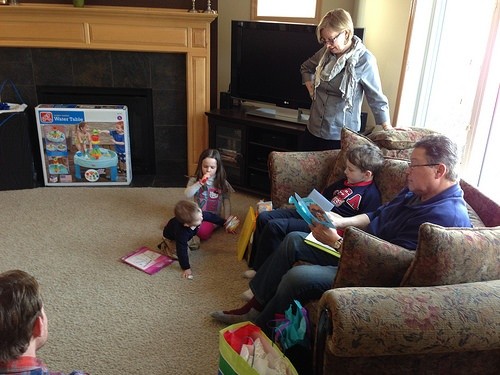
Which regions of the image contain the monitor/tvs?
[231,20,364,111]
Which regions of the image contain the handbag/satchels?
[217,320,299,375]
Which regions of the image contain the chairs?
[327,126,411,206]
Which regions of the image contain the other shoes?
[210,308,259,324]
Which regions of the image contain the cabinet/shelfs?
[0,109,40,190]
[205,106,368,197]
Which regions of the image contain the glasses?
[320,30,346,45]
[406,160,442,171]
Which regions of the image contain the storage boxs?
[35,104,132,187]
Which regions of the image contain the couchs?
[270,147,500,375]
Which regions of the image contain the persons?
[239,144,381,303]
[185,149,236,241]
[105,121,126,174]
[207,136,473,337]
[0,269,93,375]
[158,200,238,280]
[75,123,92,151]
[301,9,414,150]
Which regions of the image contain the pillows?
[329,226,414,287]
[366,127,441,149]
[403,222,500,287]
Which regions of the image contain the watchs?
[334,238,343,251]
